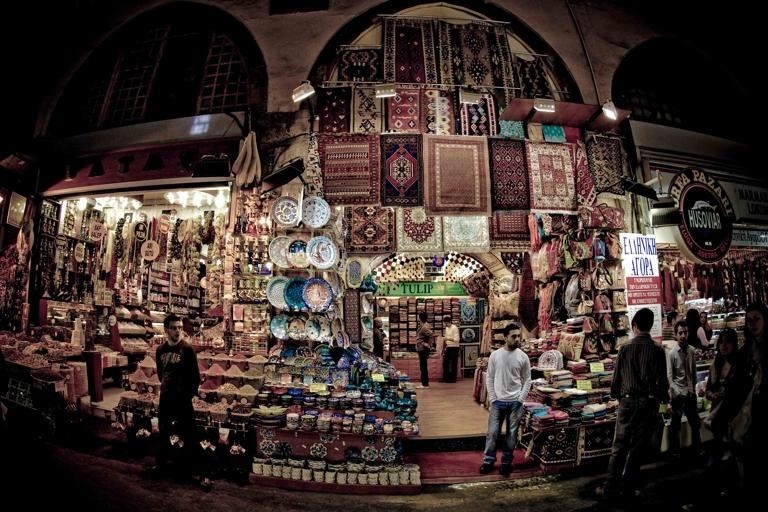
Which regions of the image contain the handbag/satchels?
[568,203,630,352]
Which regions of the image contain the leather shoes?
[480,464,511,474]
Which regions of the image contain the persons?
[414,312,439,388]
[153,313,214,492]
[478,324,532,475]
[437,317,460,382]
[375,318,387,357]
[594,303,768,511]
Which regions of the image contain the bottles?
[383,420,393,435]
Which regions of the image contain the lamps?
[59,119,242,182]
[289,79,318,105]
[648,170,678,210]
[262,156,310,190]
[597,175,660,203]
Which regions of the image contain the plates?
[305,236,337,267]
[271,196,299,227]
[268,312,372,364]
[261,364,372,388]
[537,350,564,371]
[265,277,289,310]
[301,196,329,229]
[285,239,311,268]
[267,236,294,268]
[300,278,333,312]
[282,278,306,310]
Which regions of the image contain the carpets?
[312,16,629,254]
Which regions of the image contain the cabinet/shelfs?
[1,188,633,494]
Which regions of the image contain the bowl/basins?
[286,411,374,435]
[254,414,284,428]
[255,388,378,413]
